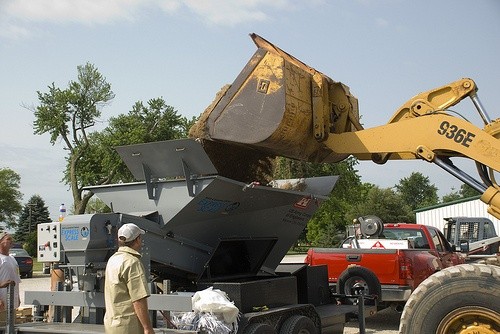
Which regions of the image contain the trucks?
[442,216,500,260]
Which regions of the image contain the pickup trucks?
[305,222,465,321]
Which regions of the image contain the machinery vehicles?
[18,138,379,334]
[199,32,500,333]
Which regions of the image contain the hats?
[117,222,146,243]
[0,232,8,240]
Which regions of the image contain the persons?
[0,232,22,334]
[103,222,155,334]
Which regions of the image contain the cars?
[9,249,34,278]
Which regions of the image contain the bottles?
[59,203,66,222]
